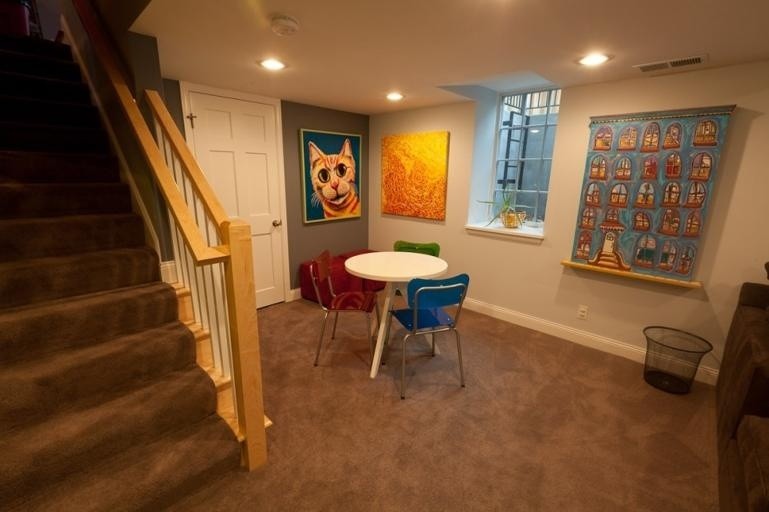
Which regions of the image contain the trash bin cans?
[643,326,713,394]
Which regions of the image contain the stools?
[299,248,388,308]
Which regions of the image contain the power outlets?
[577,304,588,320]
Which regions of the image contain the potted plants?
[485,188,531,232]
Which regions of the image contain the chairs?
[309,240,470,399]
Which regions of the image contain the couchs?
[715,281,769,512]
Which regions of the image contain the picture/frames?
[299,128,362,225]
